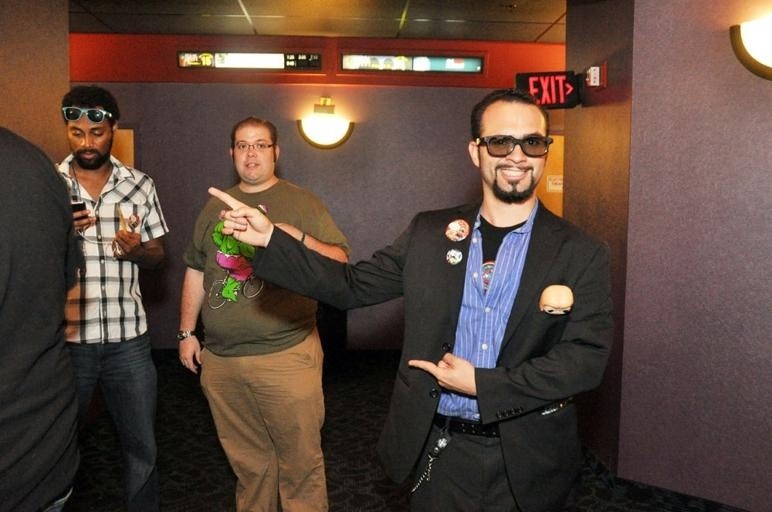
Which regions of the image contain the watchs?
[177,331,197,341]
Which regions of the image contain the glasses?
[235,141,275,151]
[476,132,554,158]
[61,106,113,123]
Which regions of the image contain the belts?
[435,414,500,438]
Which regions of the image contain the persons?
[52,85,173,511]
[177,115,350,512]
[0,126,88,512]
[208,83,614,512]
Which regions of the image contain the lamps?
[728,13,771,84]
[297,90,355,152]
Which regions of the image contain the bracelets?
[301,231,305,242]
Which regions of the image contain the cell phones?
[71,201,88,220]
